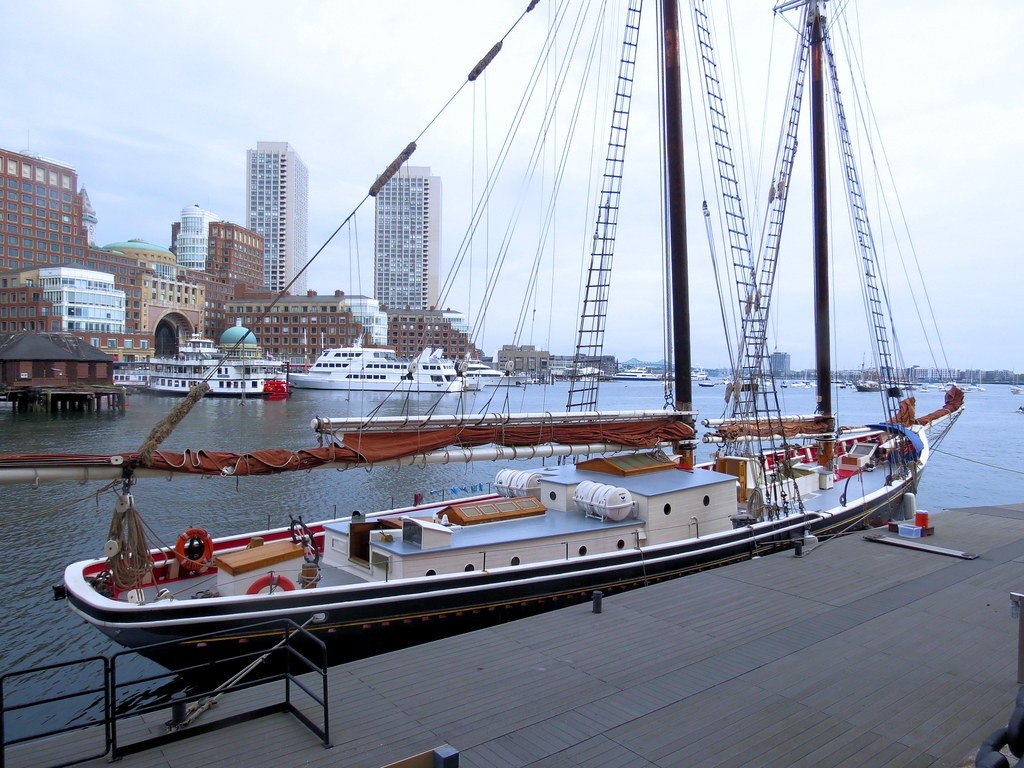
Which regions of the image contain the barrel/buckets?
[493,469,544,501]
[573,480,632,522]
[915,510,928,528]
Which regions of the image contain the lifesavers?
[245,574,296,594]
[173,527,214,570]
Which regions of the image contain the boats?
[840,378,881,392]
[698,383,716,386]
[115,332,291,398]
[286,333,531,391]
[610,368,659,381]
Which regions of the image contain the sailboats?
[52,0,929,691]
